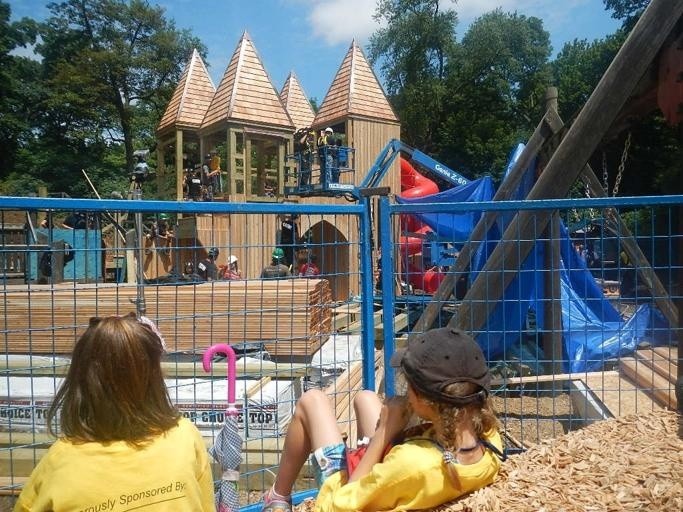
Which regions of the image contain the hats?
[227,255,238,264]
[389,327,490,407]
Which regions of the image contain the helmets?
[160,214,169,220]
[271,247,284,259]
[324,127,333,135]
[208,247,219,256]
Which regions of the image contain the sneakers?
[261,493,292,512]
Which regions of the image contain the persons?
[264,181,273,197]
[261,214,320,279]
[135,157,149,189]
[153,213,174,256]
[14,312,216,512]
[182,247,242,281]
[183,150,221,199]
[318,127,335,174]
[262,327,504,512]
[299,129,311,185]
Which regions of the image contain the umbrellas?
[203,342,243,512]
